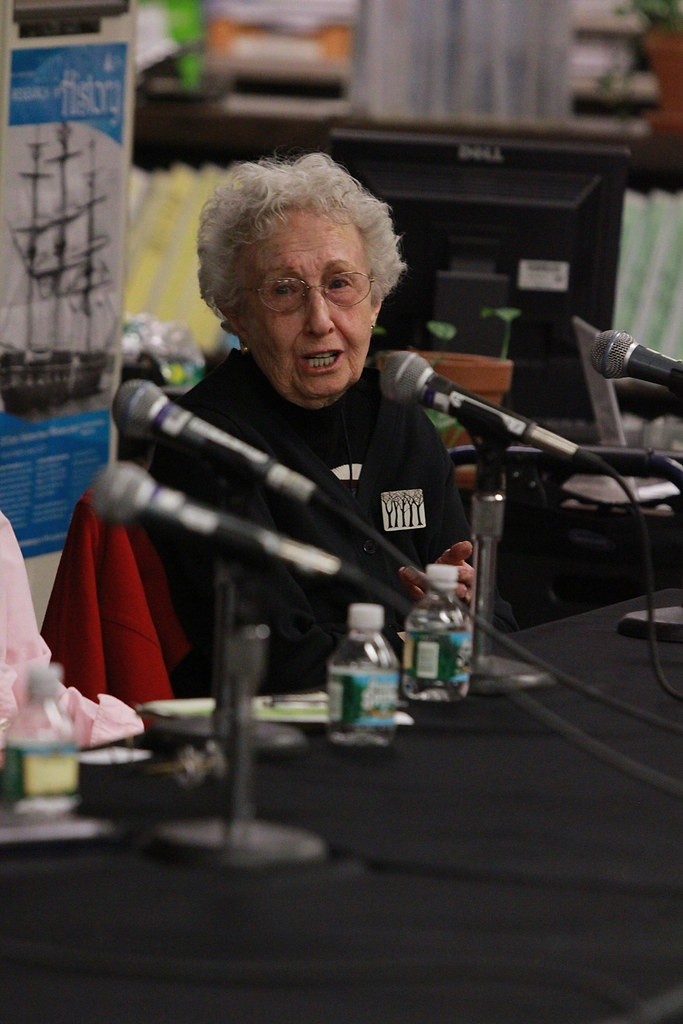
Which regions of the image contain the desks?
[0,581,683,1024]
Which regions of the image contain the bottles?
[326,603,399,748]
[402,564,473,702]
[1,664,81,820]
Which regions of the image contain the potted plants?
[370,305,522,449]
[593,0,683,142]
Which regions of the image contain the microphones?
[590,329,683,397]
[380,350,603,467]
[85,456,411,622]
[113,378,407,574]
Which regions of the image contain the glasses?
[233,270,376,313]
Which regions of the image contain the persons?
[0,512,142,748]
[144,154,477,692]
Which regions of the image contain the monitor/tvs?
[324,125,631,377]
